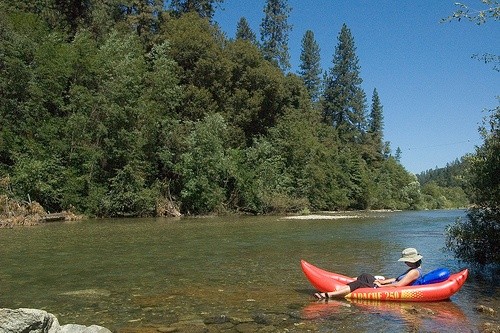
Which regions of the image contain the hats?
[398,248,423,263]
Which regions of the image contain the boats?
[300,258,468,302]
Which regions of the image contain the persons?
[314,248,423,301]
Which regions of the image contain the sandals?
[313,292,328,299]
